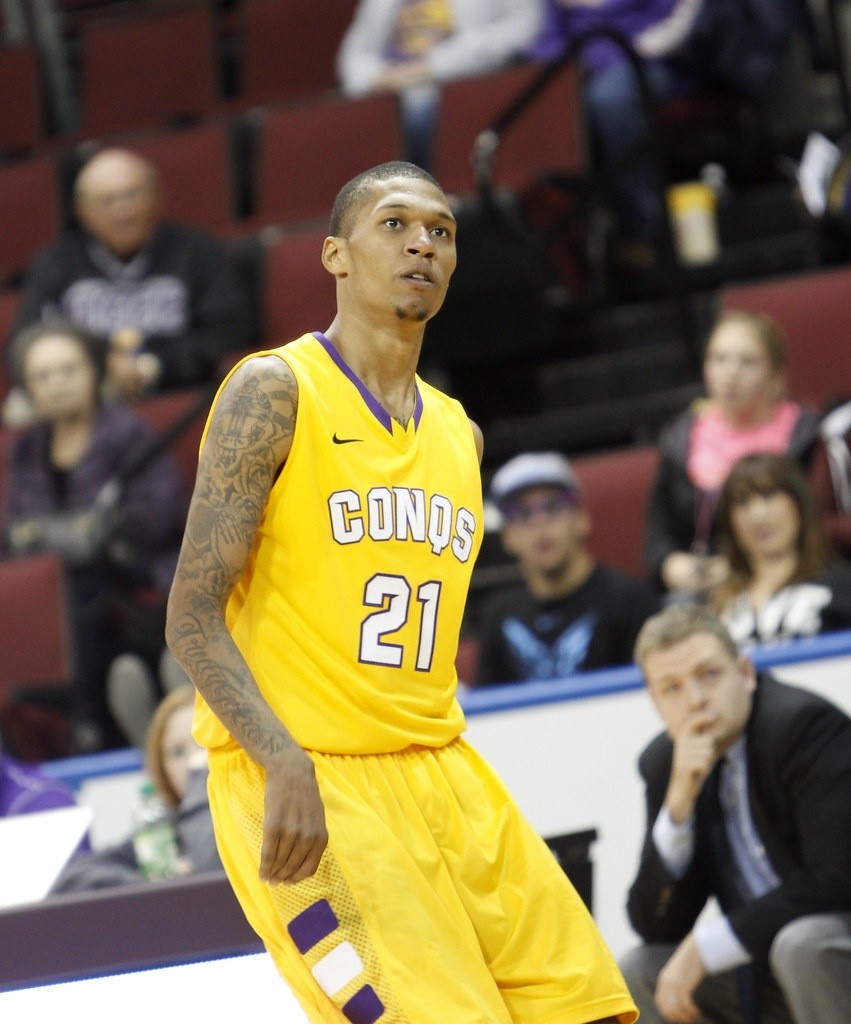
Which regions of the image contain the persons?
[624,605,851,1024]
[165,160,641,1024]
[0,0,851,901]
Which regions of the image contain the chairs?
[0,0,850,710]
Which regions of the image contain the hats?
[491,451,582,506]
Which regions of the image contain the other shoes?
[105,654,156,746]
[160,646,191,694]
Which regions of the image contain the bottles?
[131,784,178,885]
